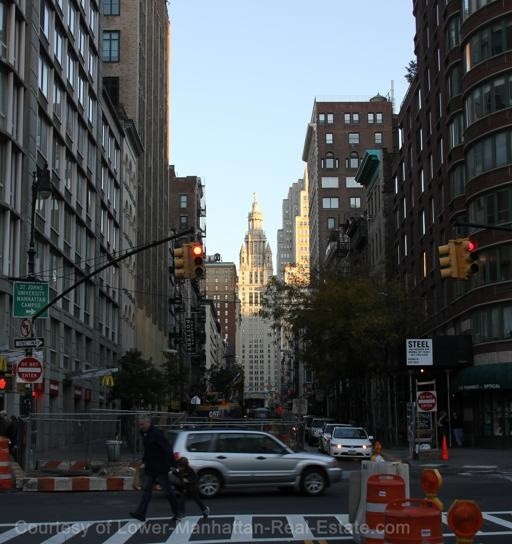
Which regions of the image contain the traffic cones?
[440,434,449,460]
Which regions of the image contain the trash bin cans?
[106,440,122,462]
[364,474,442,544]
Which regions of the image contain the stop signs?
[416,391,437,412]
[16,356,44,384]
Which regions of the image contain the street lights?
[27,166,51,276]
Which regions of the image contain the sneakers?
[130,505,210,524]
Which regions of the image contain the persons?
[438,410,448,434]
[128,413,186,524]
[0,409,29,462]
[371,439,385,463]
[170,457,210,523]
[452,411,466,448]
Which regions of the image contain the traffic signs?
[12,280,50,320]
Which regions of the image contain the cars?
[168,430,342,496]
[303,415,374,461]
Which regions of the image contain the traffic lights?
[25,390,38,399]
[174,242,205,279]
[437,238,478,281]
[0,375,12,393]
